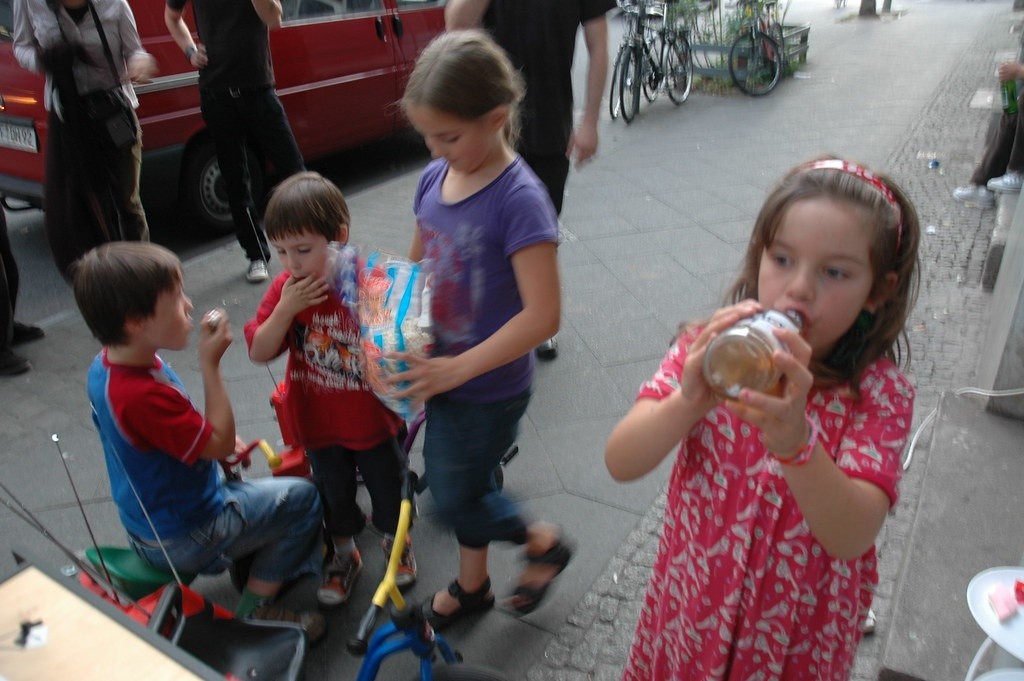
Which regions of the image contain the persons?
[10,0,158,292]
[243,170,419,591]
[400,28,574,630]
[0,202,45,377]
[604,153,919,681]
[72,239,326,645]
[162,0,307,284]
[444,0,618,360]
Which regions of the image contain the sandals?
[417,574,494,629]
[509,536,570,615]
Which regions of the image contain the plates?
[967,566,1024,662]
[975,669,1024,681]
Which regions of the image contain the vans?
[0,1,455,234]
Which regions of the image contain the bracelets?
[185,47,197,64]
[764,416,818,479]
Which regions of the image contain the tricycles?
[81,438,320,605]
[347,470,515,681]
[403,405,517,491]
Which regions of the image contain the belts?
[219,87,247,98]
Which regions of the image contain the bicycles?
[727,1,784,96]
[609,0,695,123]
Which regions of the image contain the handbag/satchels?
[76,88,138,155]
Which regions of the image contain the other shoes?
[0,352,31,376]
[9,321,45,343]
[240,604,324,639]
[535,336,557,357]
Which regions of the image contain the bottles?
[702,310,803,402]
[1001,63,1018,114]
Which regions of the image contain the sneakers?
[987,172,1024,192]
[382,533,416,587]
[953,183,993,209]
[246,259,269,282]
[317,546,363,603]
